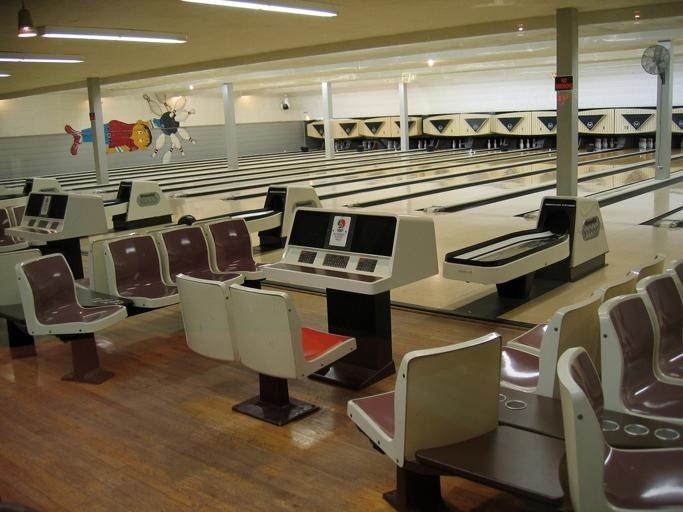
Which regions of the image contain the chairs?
[597,292,683,426]
[156,225,245,287]
[635,273,683,386]
[201,219,271,281]
[90,233,180,309]
[175,273,356,425]
[503,290,605,399]
[1,205,41,347]
[555,347,683,507]
[665,260,682,291]
[14,251,129,384]
[630,260,666,279]
[349,331,502,506]
[507,271,639,355]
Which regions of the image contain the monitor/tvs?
[25,193,68,220]
[289,210,330,248]
[351,216,397,257]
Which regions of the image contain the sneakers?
[65,125,79,136]
[70,135,81,155]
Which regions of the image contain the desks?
[414,386,566,506]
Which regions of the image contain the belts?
[107,132,111,138]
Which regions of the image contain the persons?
[65,120,152,155]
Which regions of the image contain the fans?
[642,45,670,85]
